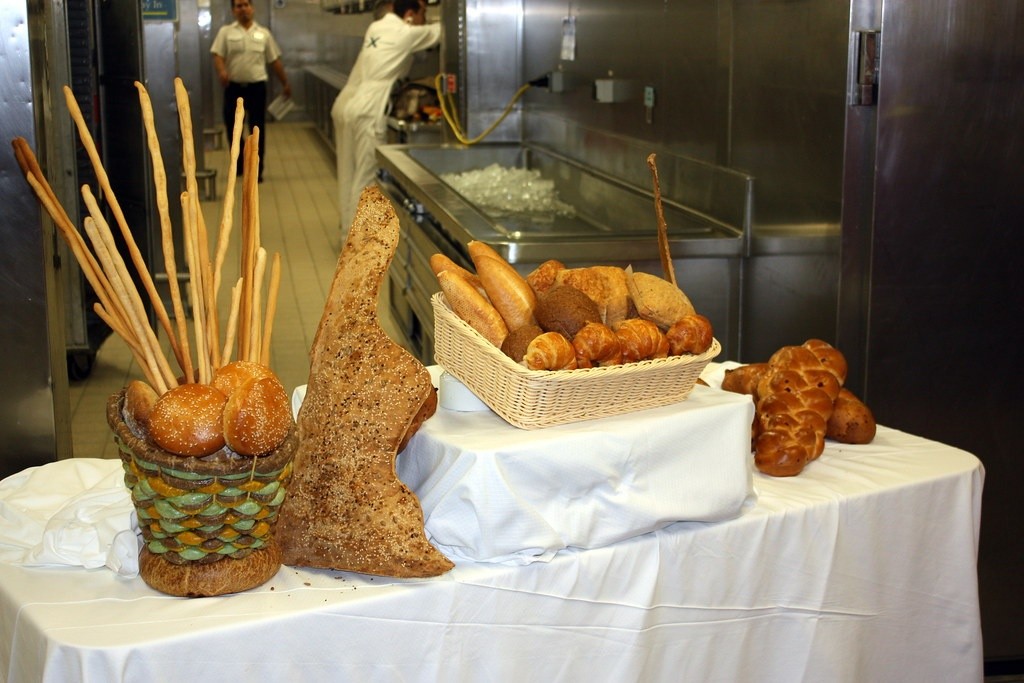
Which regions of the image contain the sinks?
[374,140,748,369]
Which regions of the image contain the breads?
[721,338,875,476]
[430,240,712,370]
[273,190,454,579]
[11,78,291,459]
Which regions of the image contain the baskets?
[432,288,723,430]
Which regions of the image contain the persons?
[331,0,442,249]
[210,0,293,182]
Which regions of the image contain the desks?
[0,433,985,683]
